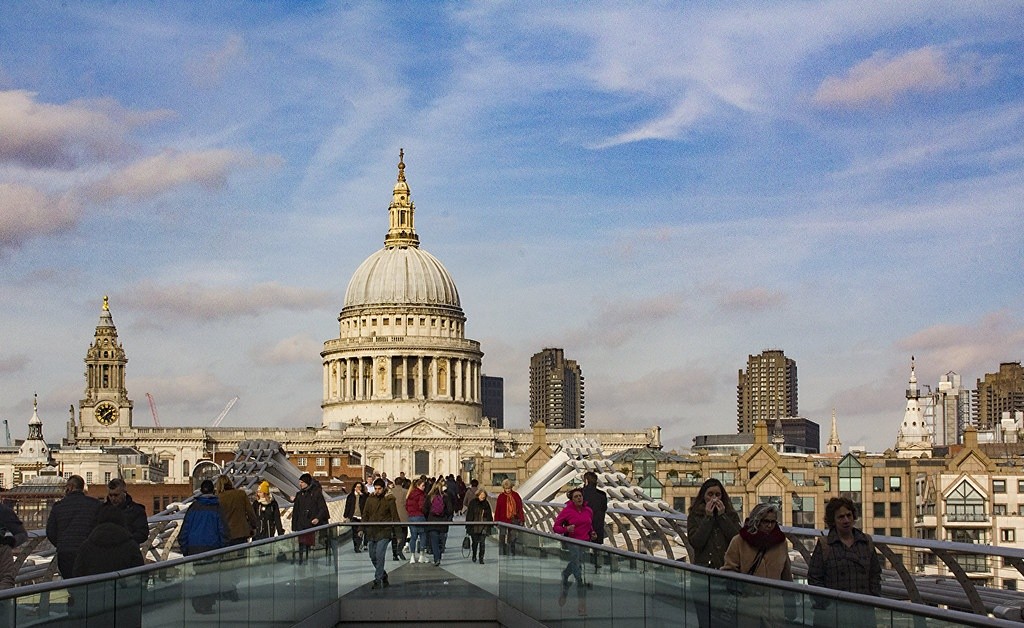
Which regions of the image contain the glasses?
[760,518,776,525]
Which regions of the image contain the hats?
[258,481,269,494]
[200,480,214,494]
[299,472,312,486]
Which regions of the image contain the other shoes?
[381,573,388,588]
[363,545,368,551]
[577,582,586,599]
[434,559,440,566]
[410,557,415,564]
[393,557,399,561]
[354,545,361,553]
[418,556,429,563]
[371,580,381,589]
[561,570,569,590]
[397,550,406,560]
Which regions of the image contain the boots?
[479,553,484,564]
[473,552,476,562]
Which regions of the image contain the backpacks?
[432,492,445,516]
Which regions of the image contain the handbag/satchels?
[463,534,470,550]
[511,514,520,524]
[726,579,752,598]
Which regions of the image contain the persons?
[580,471,608,569]
[0,503,28,619]
[344,472,466,565]
[686,478,743,628]
[178,481,228,614]
[465,489,494,564]
[808,497,882,628]
[252,480,284,556]
[463,479,479,506]
[45,474,149,628]
[494,479,526,555]
[216,475,257,601]
[720,502,797,628]
[552,488,597,588]
[291,473,329,565]
[358,478,403,589]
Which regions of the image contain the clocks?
[95,403,119,426]
